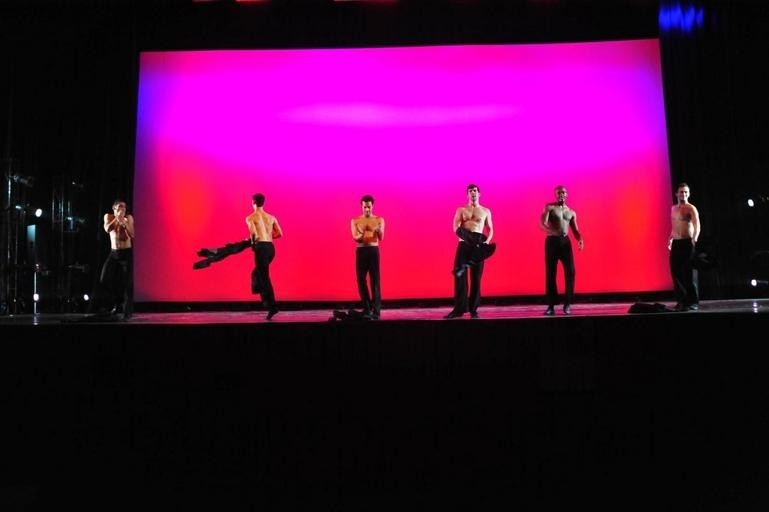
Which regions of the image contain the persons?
[665,184,703,310]
[245,193,282,320]
[444,184,496,319]
[351,195,384,317]
[539,184,583,316]
[96,201,135,322]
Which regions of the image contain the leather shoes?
[563,304,570,315]
[444,310,478,318]
[675,301,699,312]
[545,307,555,314]
